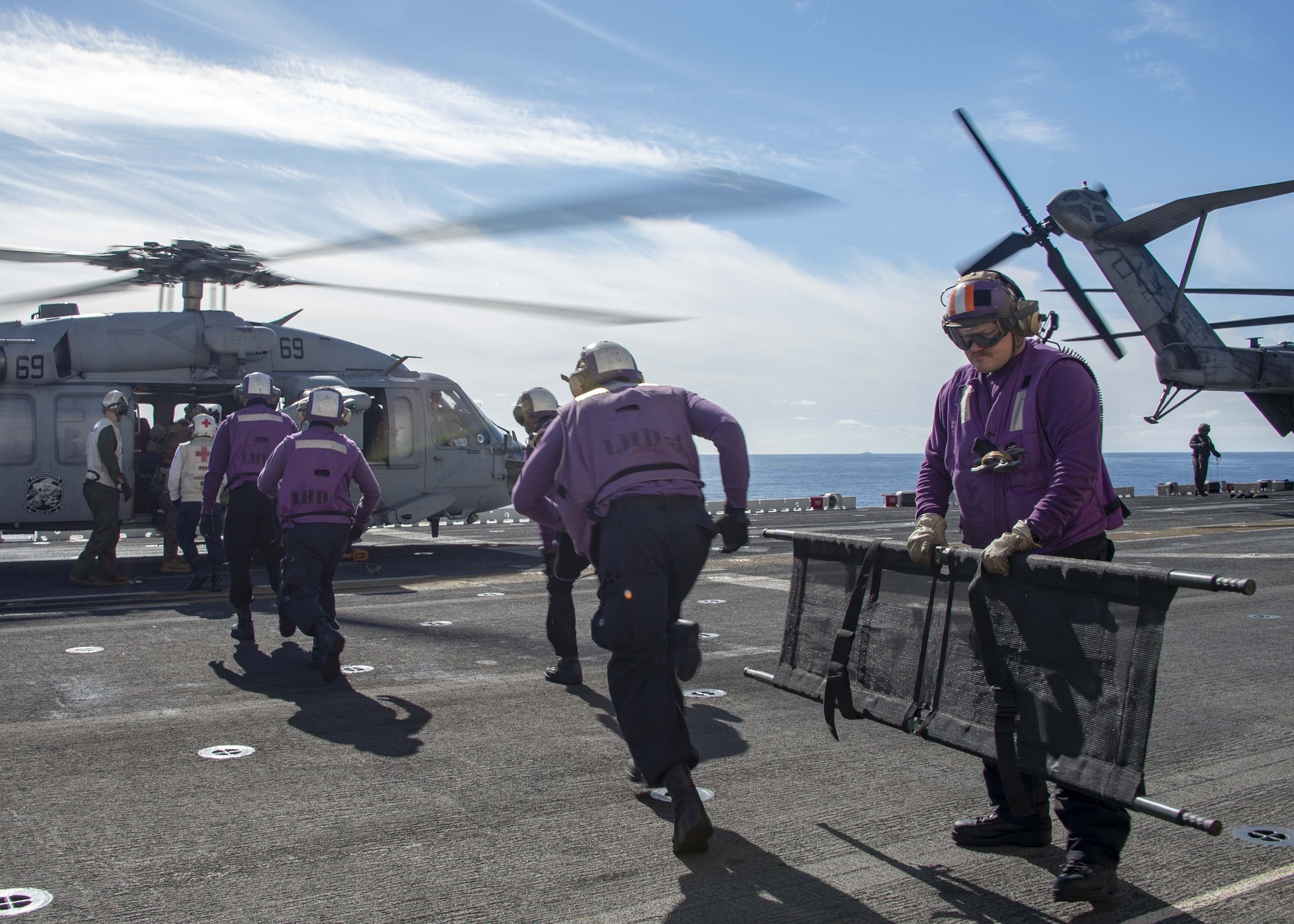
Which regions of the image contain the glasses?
[944,314,1010,351]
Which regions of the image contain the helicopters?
[0,168,843,542]
[951,104,1294,436]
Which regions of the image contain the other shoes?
[1194,490,1199,495]
[1198,492,1208,497]
[626,759,648,785]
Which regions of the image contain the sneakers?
[1053,858,1118,901]
[544,655,582,685]
[160,555,345,683]
[69,573,102,586]
[101,576,130,585]
[671,619,700,681]
[950,812,1052,847]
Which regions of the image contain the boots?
[660,763,713,854]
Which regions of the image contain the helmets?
[102,390,128,409]
[184,405,211,418]
[1198,424,1210,432]
[942,271,1016,334]
[242,372,274,400]
[307,387,344,426]
[190,413,216,437]
[561,341,644,398]
[513,387,559,436]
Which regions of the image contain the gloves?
[199,515,213,542]
[981,519,1043,576]
[907,513,949,570]
[1215,453,1221,458]
[710,505,752,554]
[121,481,132,500]
[172,498,181,509]
[1200,442,1206,447]
[350,526,362,540]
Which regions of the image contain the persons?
[200,371,296,640]
[511,341,749,856]
[68,390,132,587]
[256,387,381,683]
[513,387,700,686]
[1189,423,1220,496]
[909,270,1132,894]
[159,403,210,573]
[168,414,228,591]
